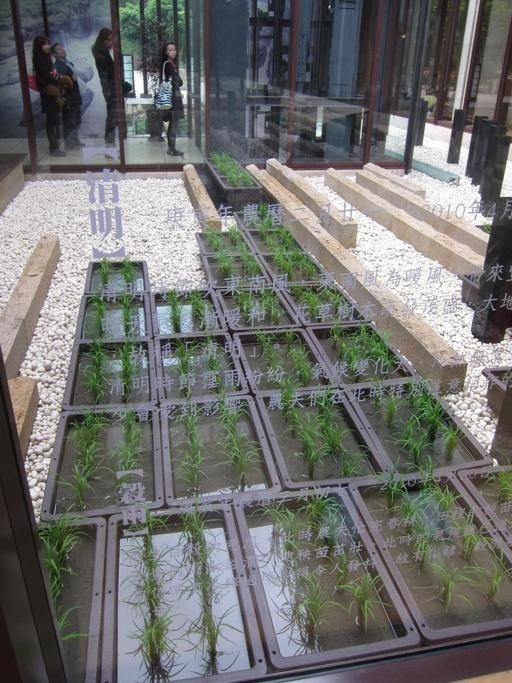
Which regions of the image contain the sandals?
[167,149,172,154]
[171,151,184,156]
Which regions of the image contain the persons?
[32,36,67,156]
[91,27,127,160]
[51,43,85,150]
[159,41,185,156]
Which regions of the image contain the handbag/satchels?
[155,61,175,110]
[26,53,40,92]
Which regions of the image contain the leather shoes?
[51,149,65,157]
